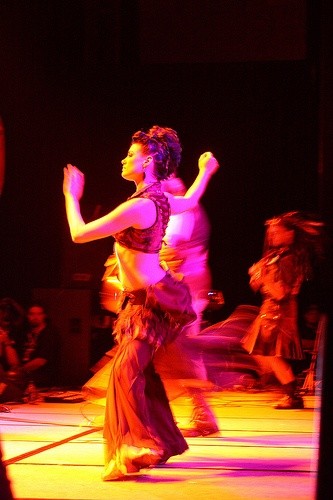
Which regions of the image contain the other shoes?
[180,421,220,438]
[102,459,124,481]
[273,393,304,410]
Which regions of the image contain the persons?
[0,172,322,435]
[63,127,219,480]
